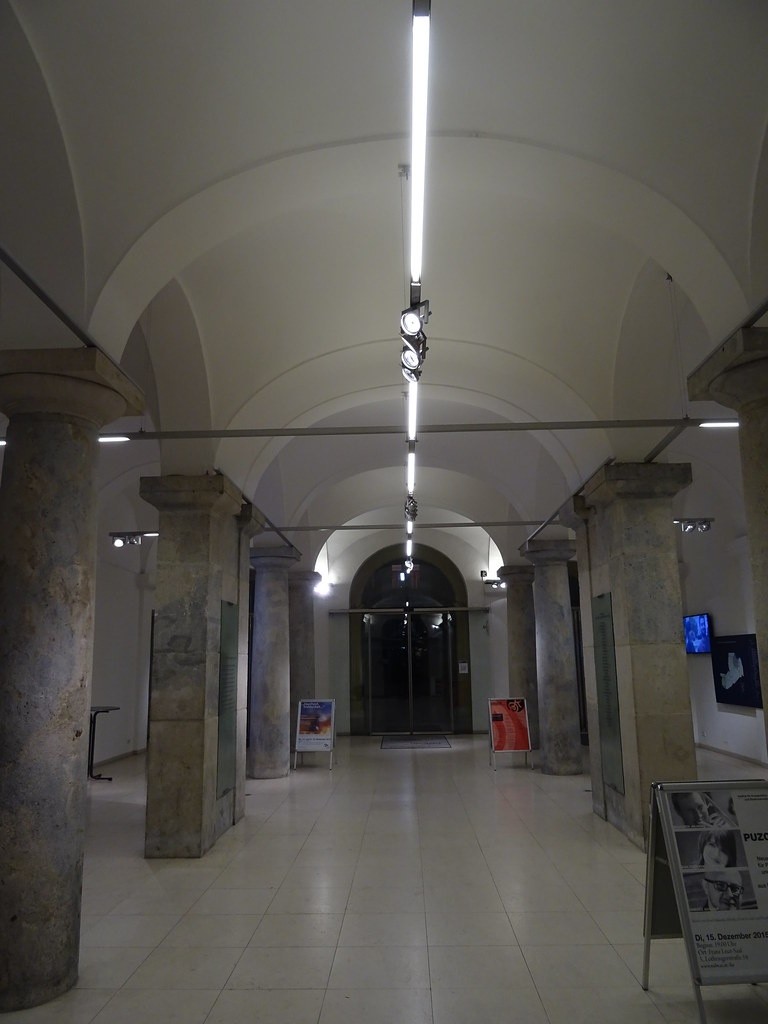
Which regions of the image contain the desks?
[90,706,120,780]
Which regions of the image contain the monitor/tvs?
[710,635,764,707]
[681,613,713,655]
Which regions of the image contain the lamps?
[401,362,422,383]
[401,346,424,370]
[401,300,432,336]
[401,330,429,359]
[484,580,506,589]
[110,530,158,547]
[674,518,716,533]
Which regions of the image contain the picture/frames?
[710,634,763,710]
